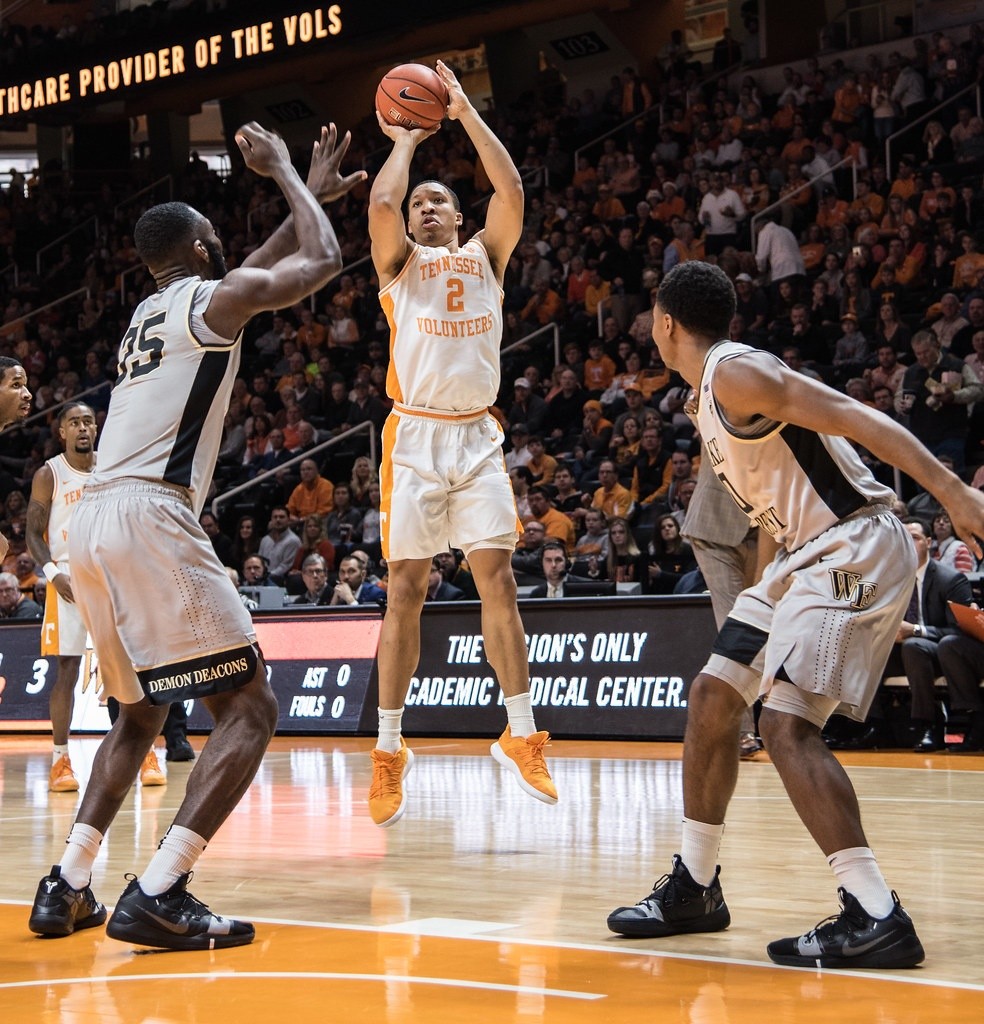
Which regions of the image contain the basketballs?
[375,63,449,131]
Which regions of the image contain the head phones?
[537,541,572,571]
[242,555,270,580]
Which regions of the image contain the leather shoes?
[738,731,761,755]
[824,724,886,750]
[950,726,984,752]
[915,724,940,750]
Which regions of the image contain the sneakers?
[165,738,196,761]
[490,723,558,806]
[28,865,106,933]
[767,887,925,968]
[608,855,730,933]
[139,752,165,787]
[368,736,415,827]
[49,753,79,791]
[106,873,255,949]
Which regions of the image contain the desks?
[0,592,720,744]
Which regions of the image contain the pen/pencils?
[336,581,341,585]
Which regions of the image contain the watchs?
[912,625,922,638]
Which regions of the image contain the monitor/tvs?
[562,580,617,597]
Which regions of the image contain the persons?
[294,554,336,606]
[241,91,590,424]
[833,517,975,753]
[638,514,697,595]
[0,432,47,615]
[930,510,977,572]
[589,517,643,583]
[368,60,558,828]
[200,375,386,592]
[242,553,279,586]
[606,259,983,969]
[502,323,983,758]
[526,16,984,325]
[0,149,243,429]
[0,573,42,621]
[26,121,367,949]
[223,566,262,610]
[329,555,386,605]
[528,541,582,599]
[424,558,466,601]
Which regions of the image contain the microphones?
[254,576,263,580]
[559,569,568,577]
